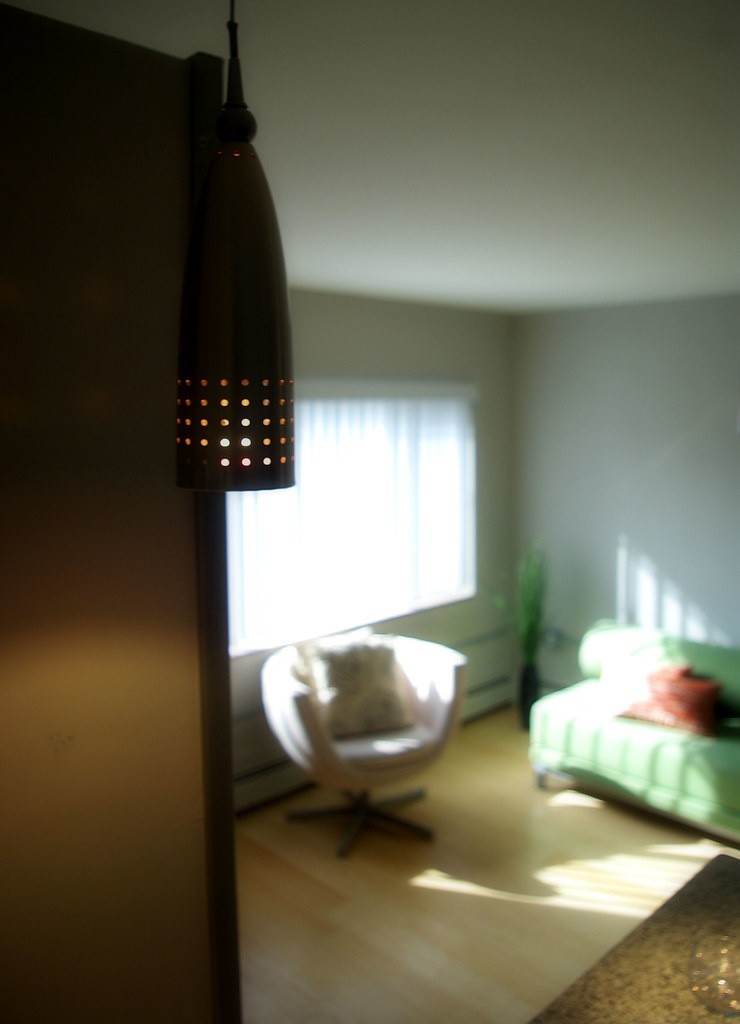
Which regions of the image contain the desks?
[525,855,740,1024]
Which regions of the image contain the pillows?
[308,637,412,739]
[614,664,723,737]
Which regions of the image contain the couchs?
[527,616,740,844]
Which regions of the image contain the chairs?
[261,628,470,861]
